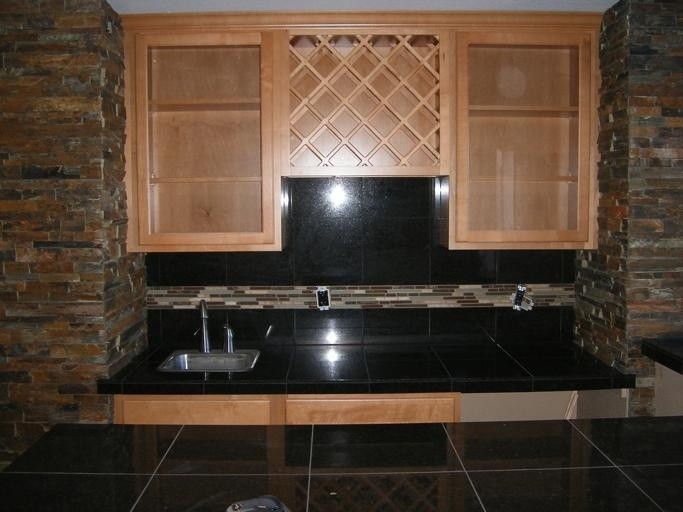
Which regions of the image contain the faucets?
[199,298,212,354]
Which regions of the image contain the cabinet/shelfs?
[131,28,292,253]
[436,38,593,246]
[287,31,452,177]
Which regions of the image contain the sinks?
[158,349,261,373]
